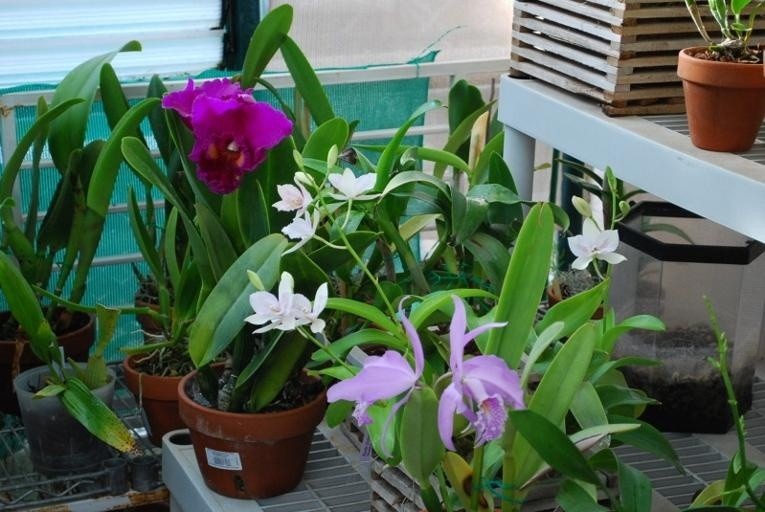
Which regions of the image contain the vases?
[123,353,330,501]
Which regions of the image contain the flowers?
[315,204,688,512]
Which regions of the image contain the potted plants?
[677,0,765,155]
[543,159,696,343]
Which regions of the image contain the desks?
[494,73,765,259]
